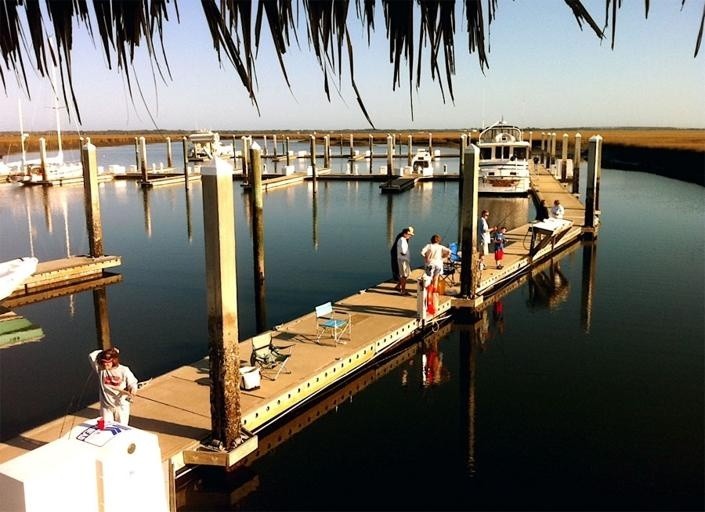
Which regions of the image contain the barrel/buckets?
[239,367,261,390]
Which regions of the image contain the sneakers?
[395,286,409,296]
[479,265,488,270]
[497,264,504,269]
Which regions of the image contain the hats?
[408,227,414,235]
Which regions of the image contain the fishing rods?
[110,386,211,419]
[489,195,529,235]
[505,238,558,244]
[439,207,462,245]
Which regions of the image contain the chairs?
[437,271,455,287]
[315,301,351,346]
[248,332,292,381]
[446,242,462,273]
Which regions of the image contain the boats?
[410,149,434,176]
[472,121,533,197]
[188,141,236,159]
[0,62,104,187]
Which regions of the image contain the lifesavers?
[428,284,439,313]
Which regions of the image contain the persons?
[492,226,510,272]
[394,228,413,297]
[477,209,499,270]
[419,233,453,292]
[89,347,140,428]
[550,200,565,220]
[389,226,415,292]
[535,198,551,242]
[532,153,540,177]
